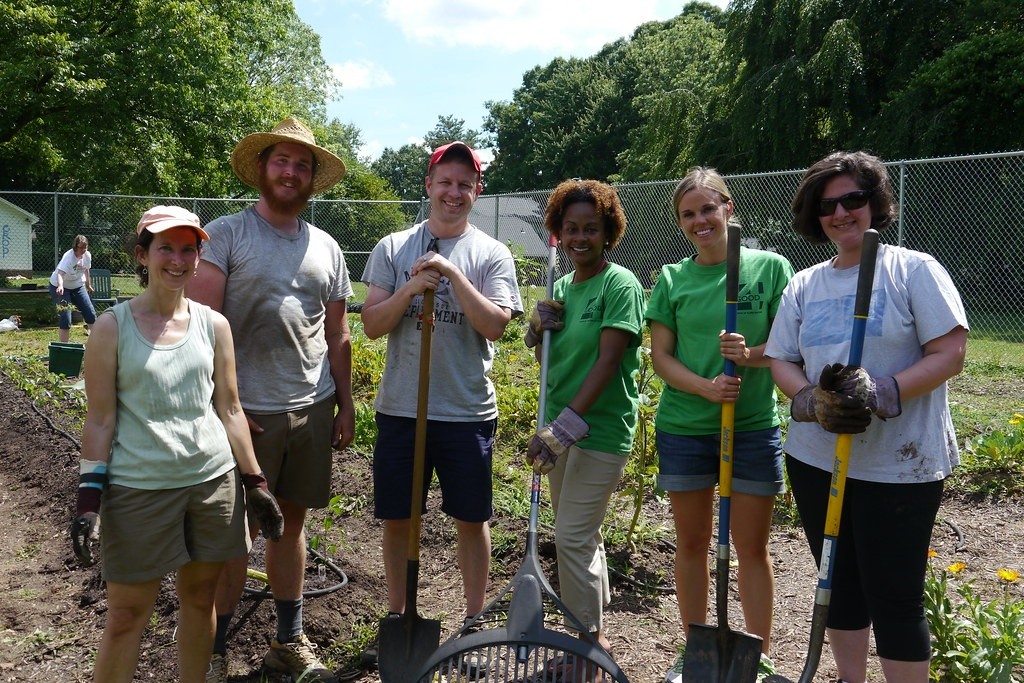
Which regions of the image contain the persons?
[523,178,644,683]
[645,164,796,683]
[48,234,98,342]
[183,117,356,683]
[68,205,286,683]
[359,141,524,677]
[761,152,969,683]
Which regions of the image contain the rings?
[423,258,427,260]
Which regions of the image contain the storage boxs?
[48,340,85,377]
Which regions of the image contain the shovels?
[378,290,442,683]
[681,224,762,683]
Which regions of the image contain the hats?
[231,117,346,194]
[428,141,482,184]
[136,206,211,241]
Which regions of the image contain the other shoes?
[754,652,777,683]
[663,635,687,683]
[360,611,404,663]
[460,616,494,674]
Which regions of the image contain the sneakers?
[262,631,337,683]
[206,651,229,683]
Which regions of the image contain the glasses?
[809,188,876,218]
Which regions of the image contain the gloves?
[242,472,284,542]
[70,459,108,567]
[789,364,872,434]
[827,362,901,422]
[525,406,592,475]
[524,297,566,348]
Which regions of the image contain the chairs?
[87,269,118,310]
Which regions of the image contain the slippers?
[546,650,613,677]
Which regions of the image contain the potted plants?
[68,306,84,324]
